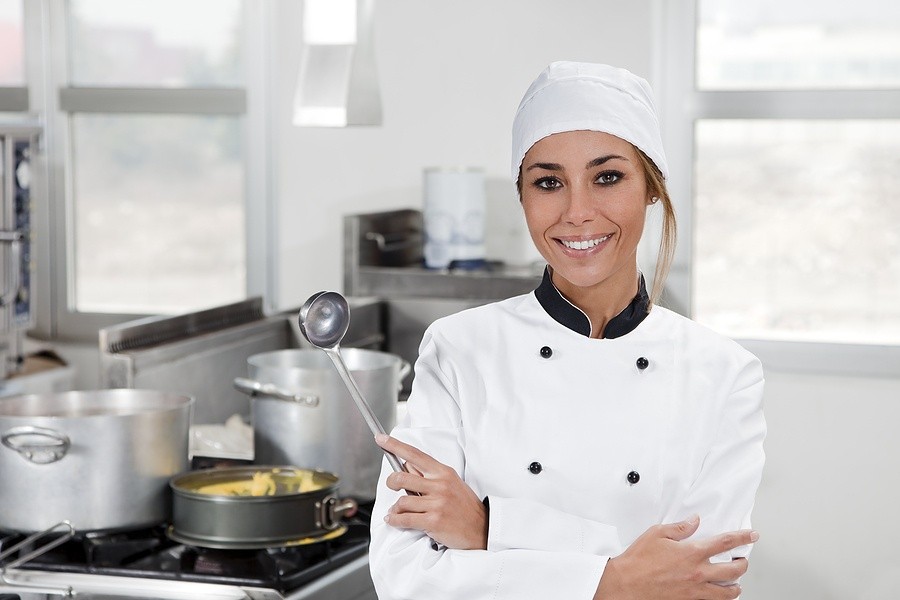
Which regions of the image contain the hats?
[511,60,668,204]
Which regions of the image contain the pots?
[0,347,411,548]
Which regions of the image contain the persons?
[366,60,767,600]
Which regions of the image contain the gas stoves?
[0,454,381,600]
